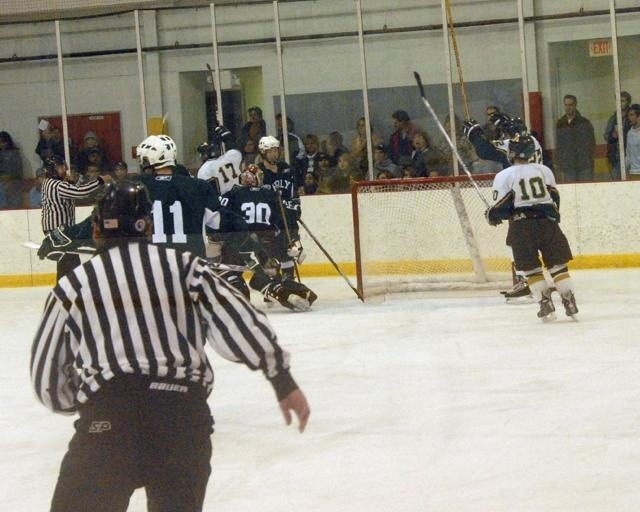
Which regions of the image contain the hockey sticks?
[206,63,231,154]
[161,110,171,134]
[414,71,491,207]
[297,217,386,306]
[22,240,294,271]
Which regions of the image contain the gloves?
[462,120,479,135]
[286,246,300,258]
[37,236,54,260]
[215,127,226,138]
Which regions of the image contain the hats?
[84,133,98,140]
[112,161,127,168]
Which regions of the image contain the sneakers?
[505,286,530,297]
[287,294,311,311]
[537,300,555,318]
[562,295,578,315]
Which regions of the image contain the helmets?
[197,141,220,157]
[91,180,154,237]
[135,135,178,169]
[490,114,510,130]
[508,139,535,160]
[239,164,264,188]
[258,137,281,154]
[502,118,526,137]
[45,156,63,180]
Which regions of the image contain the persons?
[30,179,312,512]
[1,132,23,209]
[36,106,320,313]
[445,106,579,318]
[300,110,447,195]
[555,95,596,182]
[604,90,640,181]
